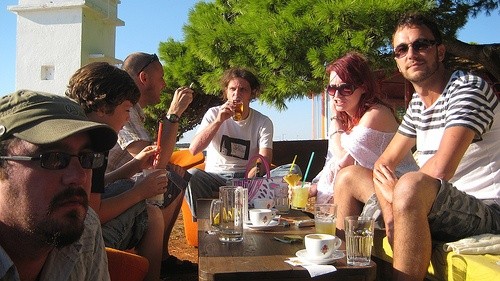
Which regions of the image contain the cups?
[232,99,244,121]
[314,203,337,236]
[304,233,342,259]
[344,215,376,267]
[143,169,166,207]
[209,185,245,243]
[274,185,291,215]
[282,171,302,186]
[289,182,311,211]
[248,209,273,226]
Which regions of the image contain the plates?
[245,220,280,230]
[295,249,346,265]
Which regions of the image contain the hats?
[0,90,118,154]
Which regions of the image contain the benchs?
[373,229,500,281]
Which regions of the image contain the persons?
[184,69,303,222]
[0,51,193,281]
[333,10,500,281]
[287,53,420,215]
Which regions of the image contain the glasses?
[0,151,108,171]
[392,38,440,59]
[324,83,359,96]
[136,53,159,77]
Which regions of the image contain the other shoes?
[160,255,198,280]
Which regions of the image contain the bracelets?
[330,116,342,121]
[328,130,344,139]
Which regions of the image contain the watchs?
[162,113,181,123]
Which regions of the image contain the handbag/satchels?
[234,154,289,212]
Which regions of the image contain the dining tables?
[196,198,377,281]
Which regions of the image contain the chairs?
[105,147,277,281]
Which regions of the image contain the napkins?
[284,256,338,278]
